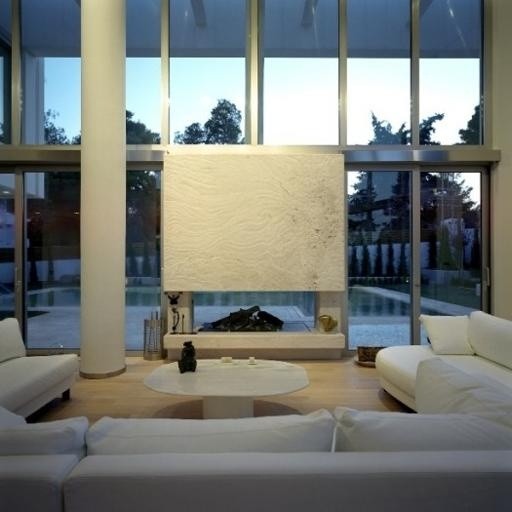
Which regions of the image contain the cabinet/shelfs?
[163,328,346,361]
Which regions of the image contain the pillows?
[418,314,477,356]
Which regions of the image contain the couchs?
[0,318,80,419]
[1,407,512,510]
[375,311,512,413]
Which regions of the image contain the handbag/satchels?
[212,306,283,331]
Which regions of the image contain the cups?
[249,357,255,364]
[221,357,232,363]
